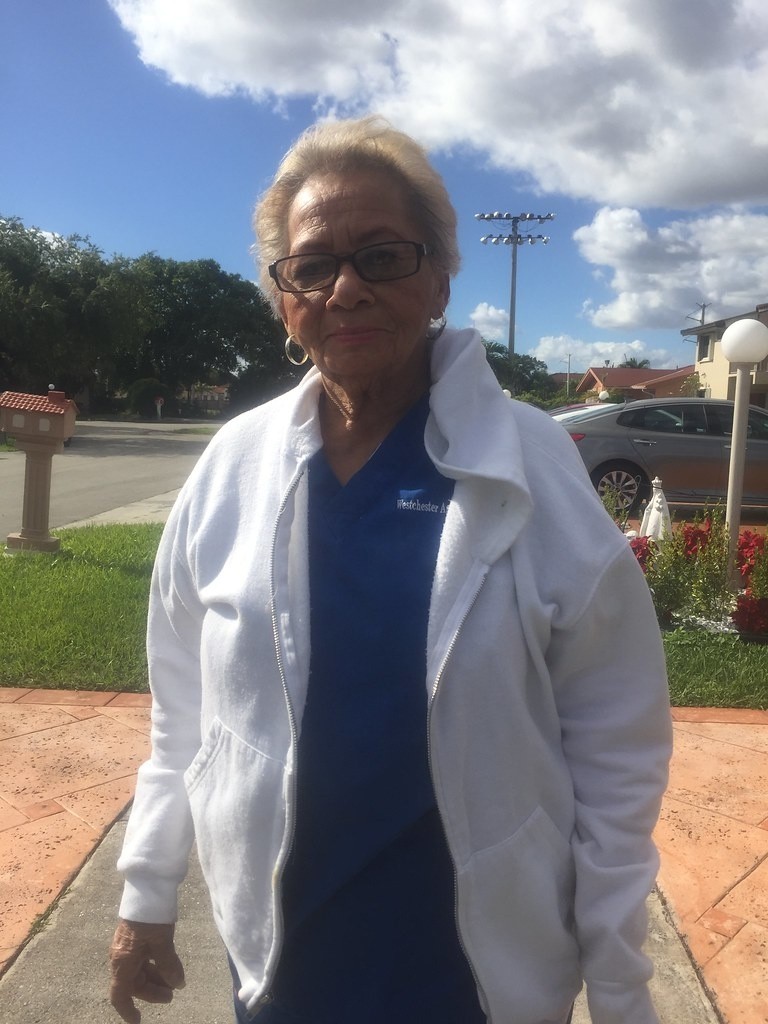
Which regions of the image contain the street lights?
[473,212,558,384]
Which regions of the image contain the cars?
[537,398,768,518]
[549,403,735,436]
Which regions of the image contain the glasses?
[268,240,434,293]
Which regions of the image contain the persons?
[640,476,673,542]
[109,117,672,1024]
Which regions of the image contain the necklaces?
[320,371,422,431]
